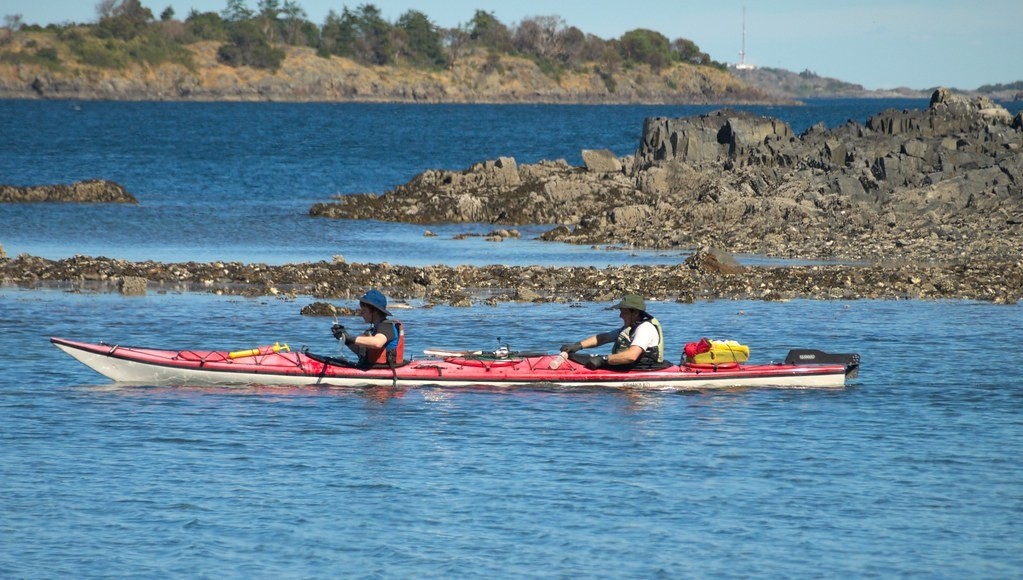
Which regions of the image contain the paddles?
[423,348,549,359]
[331,314,346,351]
[178,342,291,361]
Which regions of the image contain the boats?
[49,337,860,391]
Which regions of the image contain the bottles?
[550,352,570,370]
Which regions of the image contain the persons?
[332,289,404,366]
[559,293,665,368]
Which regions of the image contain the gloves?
[330,324,356,345]
[584,355,608,370]
[560,341,583,353]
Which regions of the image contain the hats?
[611,294,654,319]
[354,289,393,316]
